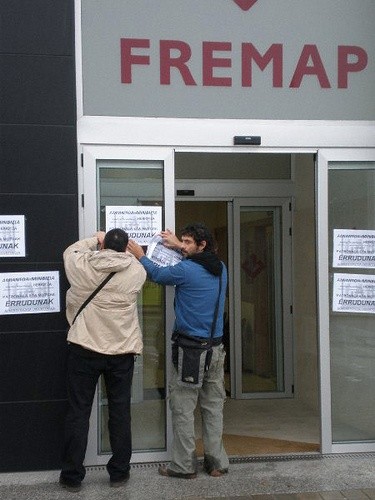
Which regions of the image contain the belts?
[213,338,223,346]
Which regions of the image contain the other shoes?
[205,464,229,476]
[111,474,130,487]
[59,475,81,492]
[158,467,197,479]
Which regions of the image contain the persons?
[126,224,230,478]
[59,229,147,492]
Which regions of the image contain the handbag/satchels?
[62,328,96,361]
[171,331,213,385]
